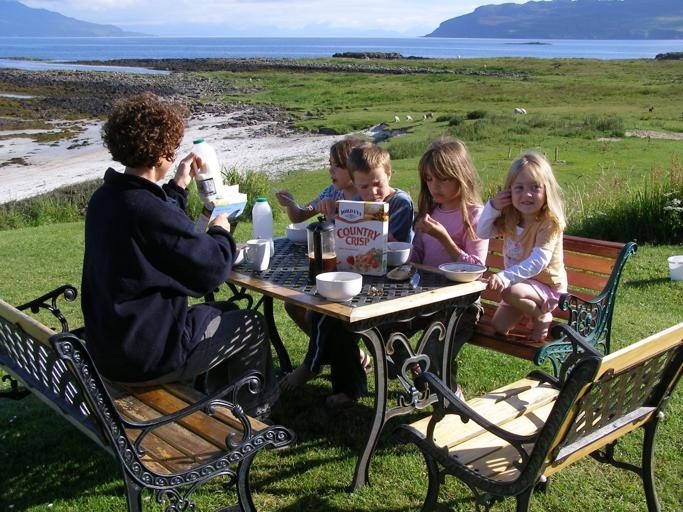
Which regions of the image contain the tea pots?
[305,216,337,285]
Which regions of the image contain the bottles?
[407,271,420,289]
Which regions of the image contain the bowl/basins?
[231,244,251,265]
[381,242,414,267]
[315,271,365,302]
[437,261,486,283]
[284,222,309,245]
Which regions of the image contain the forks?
[272,186,311,214]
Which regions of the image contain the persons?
[277,146,416,406]
[411,139,490,424]
[275,139,373,375]
[81,91,284,423]
[476,153,568,342]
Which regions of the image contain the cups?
[242,238,273,273]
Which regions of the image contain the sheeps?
[649,107,653,112]
[405,116,412,121]
[514,108,526,114]
[422,113,433,121]
[394,116,400,122]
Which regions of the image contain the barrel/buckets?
[191,139,225,204]
[668,256,683,281]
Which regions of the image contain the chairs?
[399,324,683,508]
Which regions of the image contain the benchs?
[0,283,296,512]
[410,209,639,381]
